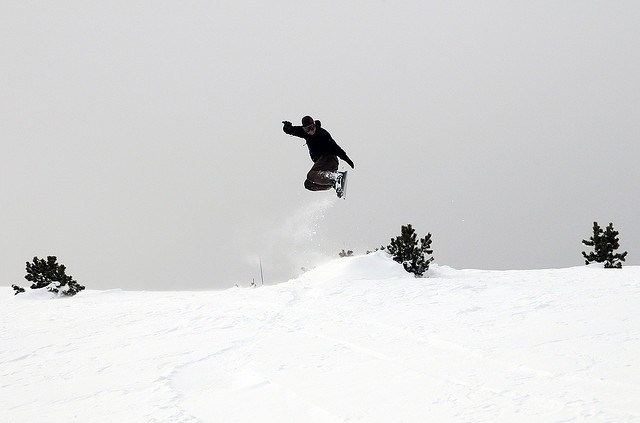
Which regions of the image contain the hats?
[302,116,314,126]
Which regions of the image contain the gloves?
[281,121,294,130]
[343,156,355,169]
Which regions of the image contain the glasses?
[302,123,314,131]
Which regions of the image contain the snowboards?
[343,171,347,199]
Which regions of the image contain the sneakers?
[334,171,344,189]
[333,185,342,198]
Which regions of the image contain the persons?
[281,114,355,200]
